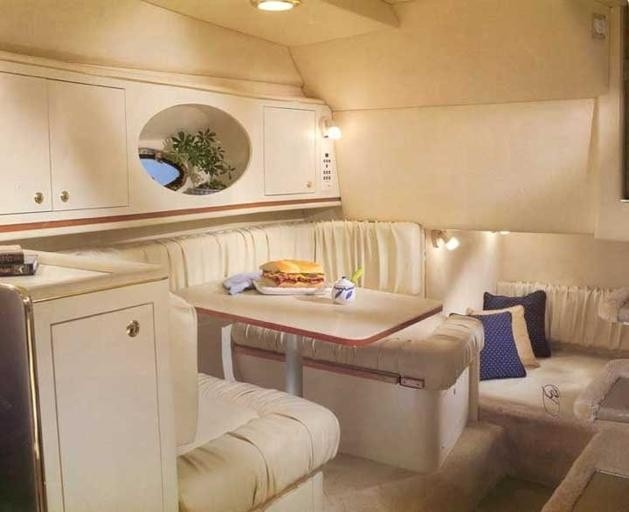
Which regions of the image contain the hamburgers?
[256,260,326,288]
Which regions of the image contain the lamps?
[431,230,460,250]
[319,116,340,139]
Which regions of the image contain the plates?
[253,279,334,294]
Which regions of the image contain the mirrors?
[138,105,251,195]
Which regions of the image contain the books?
[0,252,42,276]
[0,244,26,264]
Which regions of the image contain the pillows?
[464,289,551,379]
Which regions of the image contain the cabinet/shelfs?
[263,97,317,213]
[0,51,129,242]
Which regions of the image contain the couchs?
[425,232,629,489]
[57,219,484,512]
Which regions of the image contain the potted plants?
[163,126,236,194]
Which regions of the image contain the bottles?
[330,276,356,304]
